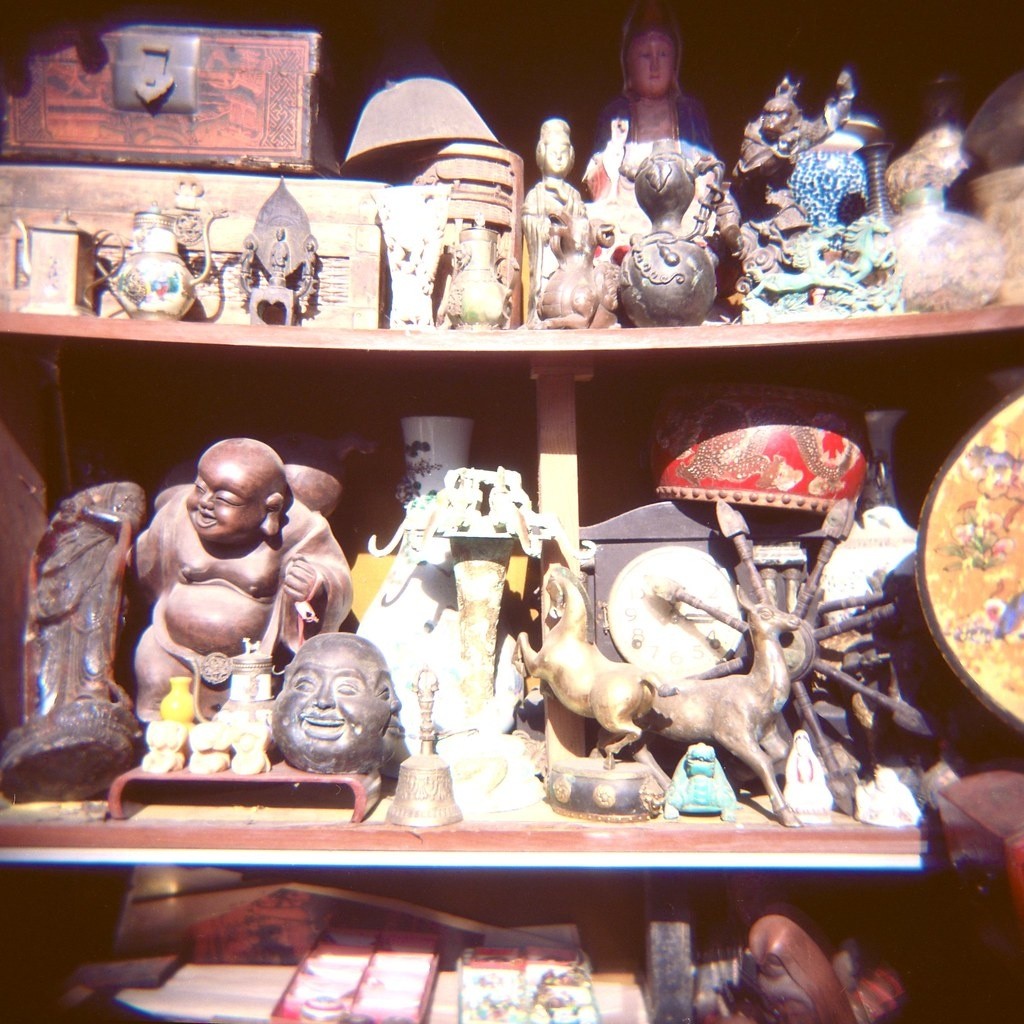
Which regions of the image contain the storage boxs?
[0,165,396,331]
[0,17,346,180]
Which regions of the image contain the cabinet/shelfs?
[0,305,1024,869]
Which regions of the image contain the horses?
[510,562,682,768]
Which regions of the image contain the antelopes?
[596,584,807,829]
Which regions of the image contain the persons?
[271,634,399,777]
[579,0,740,241]
[124,439,353,723]
[521,118,592,328]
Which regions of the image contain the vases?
[353,410,515,746]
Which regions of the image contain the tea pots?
[93,201,228,319]
[11,209,124,315]
